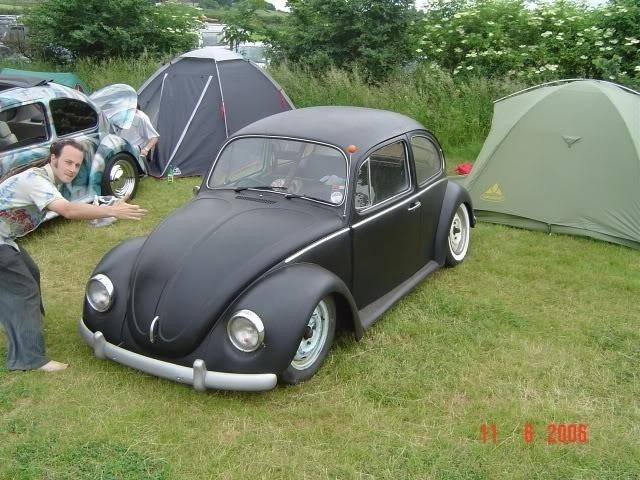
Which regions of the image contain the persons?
[109,101,160,177]
[0,137,148,373]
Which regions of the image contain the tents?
[465,77,640,252]
[0,66,89,98]
[133,46,295,179]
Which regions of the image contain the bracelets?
[144,147,149,152]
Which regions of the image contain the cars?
[79,105,477,392]
[0,82,139,223]
[197,25,272,69]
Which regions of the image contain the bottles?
[167,164,174,185]
